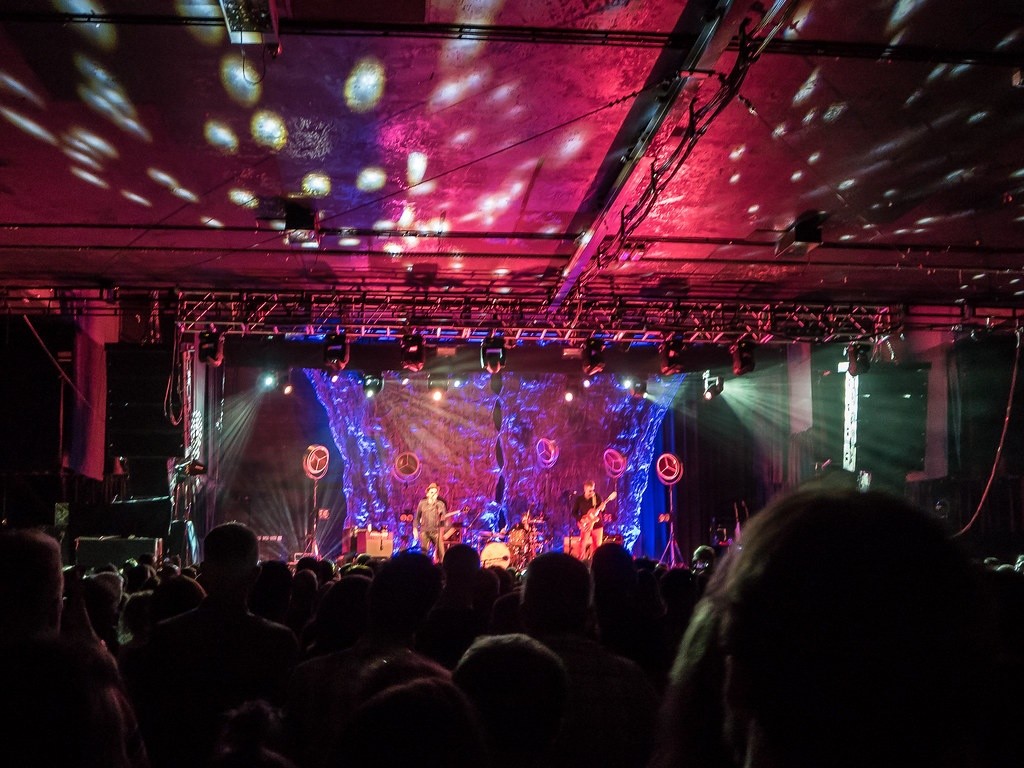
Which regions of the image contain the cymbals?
[530,531,543,535]
[529,519,544,523]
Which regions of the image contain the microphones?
[380,539,383,550]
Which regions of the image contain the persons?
[0,485,1024,768]
[412,483,446,563]
[572,480,606,559]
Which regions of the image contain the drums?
[508,529,525,547]
[480,541,511,569]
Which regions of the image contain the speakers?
[74,537,163,573]
[366,532,393,559]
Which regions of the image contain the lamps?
[199,333,225,367]
[427,373,448,400]
[478,337,507,375]
[848,340,870,377]
[398,336,425,373]
[361,368,385,398]
[319,336,350,371]
[578,337,755,401]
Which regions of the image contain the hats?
[424,483,441,493]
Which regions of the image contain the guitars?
[419,506,471,523]
[577,490,617,532]
